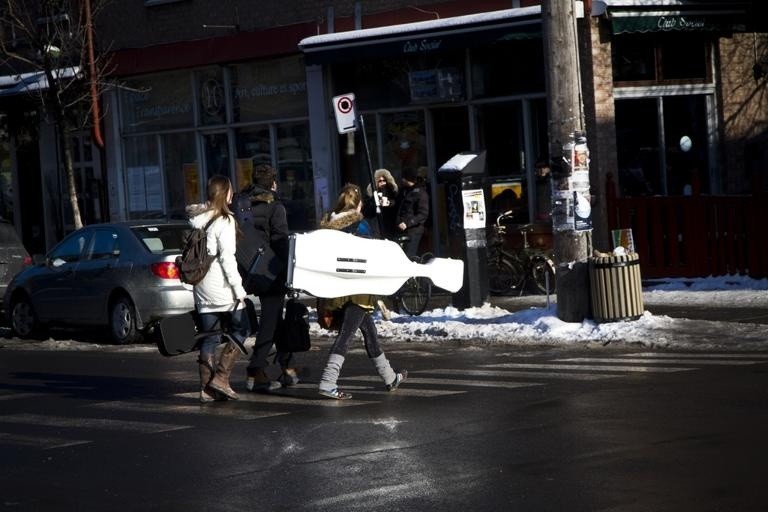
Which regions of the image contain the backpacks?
[175,212,221,284]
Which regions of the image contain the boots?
[199,340,241,402]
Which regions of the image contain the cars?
[0,218,198,346]
[261,160,313,199]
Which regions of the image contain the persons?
[236,162,309,392]
[186,175,250,403]
[362,149,430,263]
[318,182,409,401]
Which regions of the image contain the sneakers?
[319,389,352,400]
[247,371,282,390]
[284,368,298,385]
[385,369,407,392]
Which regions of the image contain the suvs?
[0,216,35,302]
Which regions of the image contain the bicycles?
[486,209,557,296]
[393,235,434,318]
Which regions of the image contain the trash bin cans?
[589,251,644,324]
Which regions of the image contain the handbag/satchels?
[278,298,311,354]
